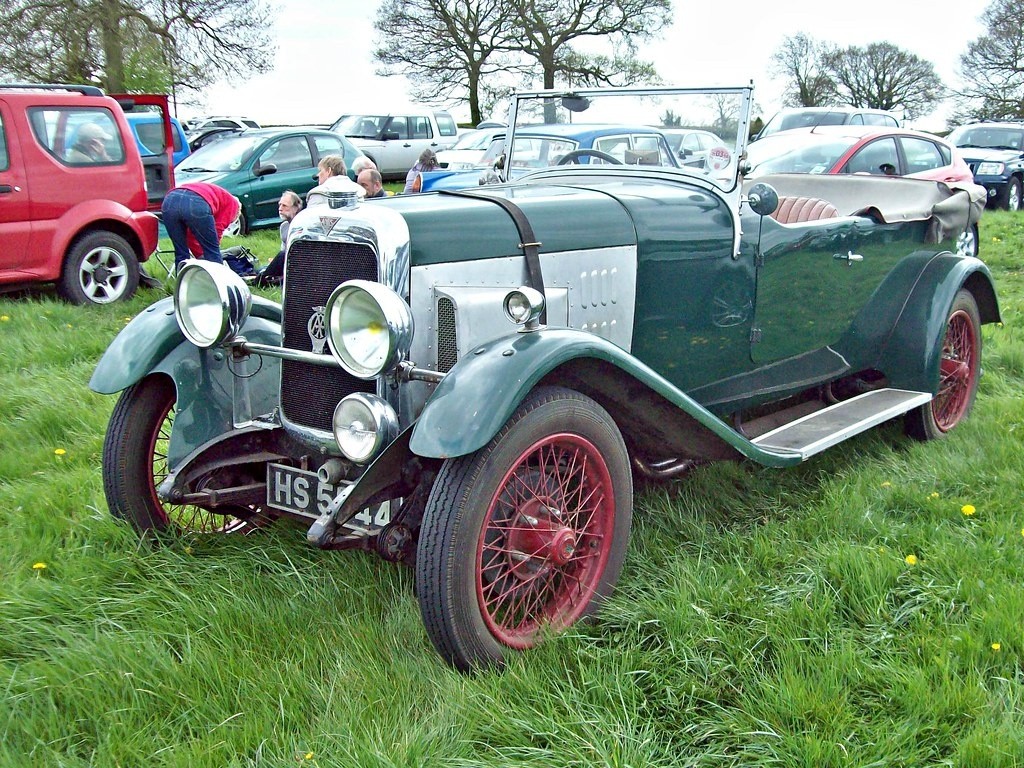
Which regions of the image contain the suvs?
[412,124,686,193]
[945,119,1024,212]
[416,128,517,173]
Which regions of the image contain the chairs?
[155,246,261,282]
[772,197,837,226]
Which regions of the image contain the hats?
[77,123,112,142]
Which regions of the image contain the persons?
[161,182,243,276]
[258,188,303,284]
[349,155,388,197]
[65,122,119,163]
[307,153,367,208]
[403,148,444,193]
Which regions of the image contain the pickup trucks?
[0,83,178,310]
[56,113,191,195]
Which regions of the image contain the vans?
[329,114,460,181]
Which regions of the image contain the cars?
[157,129,371,241]
[750,108,903,145]
[90,70,1003,679]
[179,116,260,147]
[608,130,727,174]
[704,125,983,258]
